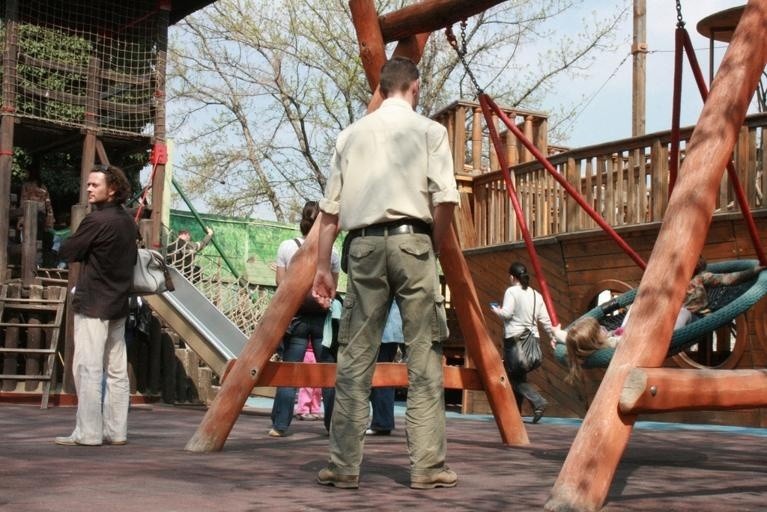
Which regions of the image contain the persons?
[605,254,767,319]
[310,54,462,492]
[562,308,695,387]
[168,224,213,282]
[488,259,557,427]
[53,163,141,448]
[260,200,406,440]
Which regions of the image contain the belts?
[353,224,423,237]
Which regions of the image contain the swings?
[443,0,767,369]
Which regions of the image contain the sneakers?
[317,469,360,489]
[533,405,546,423]
[411,468,458,490]
[55,434,78,446]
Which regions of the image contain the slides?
[141,265,250,377]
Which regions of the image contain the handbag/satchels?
[131,247,175,296]
[503,330,544,379]
